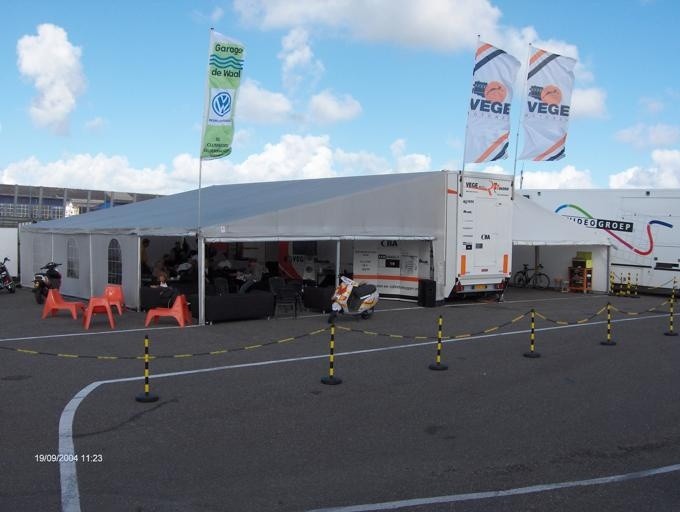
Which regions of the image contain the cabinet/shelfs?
[568,266,592,295]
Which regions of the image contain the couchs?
[141,283,274,328]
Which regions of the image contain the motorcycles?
[0,257,15,293]
[327,275,380,324]
[32,261,62,304]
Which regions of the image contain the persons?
[141,238,263,295]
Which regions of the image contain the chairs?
[42,284,123,331]
[270,275,303,321]
[305,283,335,314]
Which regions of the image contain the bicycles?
[513,263,550,291]
